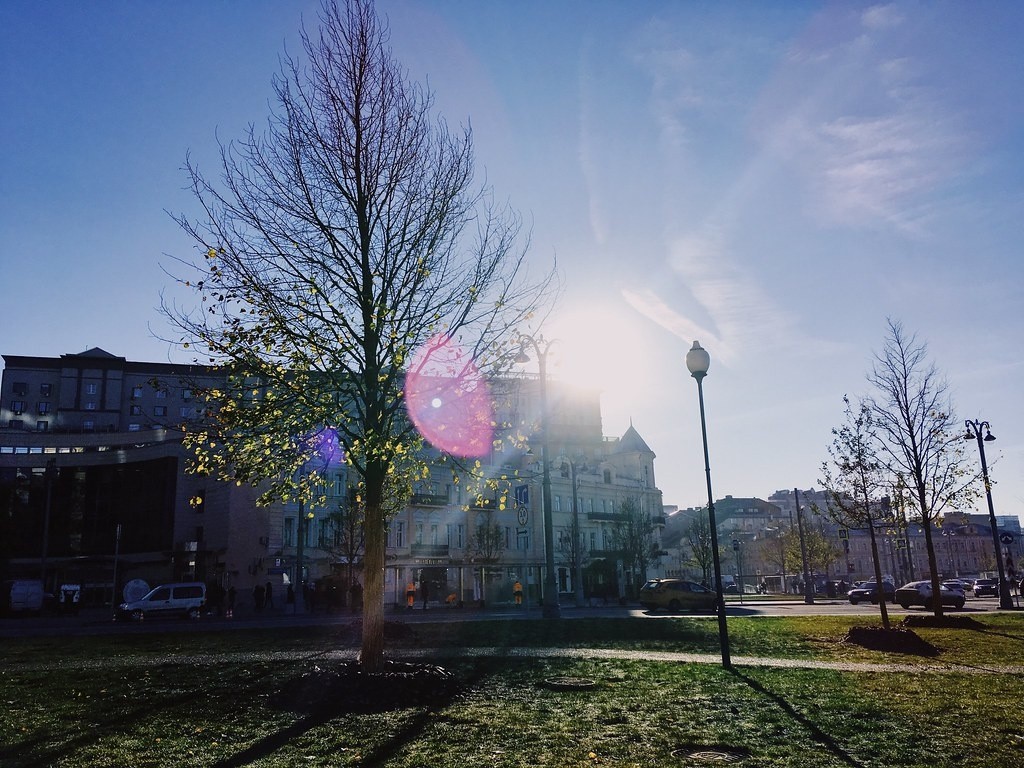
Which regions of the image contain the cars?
[894,581,966,611]
[725,585,744,593]
[946,579,972,591]
[943,583,965,595]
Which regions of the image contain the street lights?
[515,332,570,616]
[963,419,1014,608]
[684,340,734,667]
[941,531,959,578]
[553,454,595,607]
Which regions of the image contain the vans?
[0,579,44,614]
[116,580,208,621]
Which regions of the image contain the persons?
[513,581,522,605]
[405,582,416,608]
[445,594,456,604]
[229,587,235,608]
[254,582,274,607]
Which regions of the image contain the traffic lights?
[733,539,738,549]
[843,540,848,546]
[896,538,907,548]
[837,529,848,540]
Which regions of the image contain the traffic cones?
[226,608,232,620]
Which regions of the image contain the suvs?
[973,578,998,597]
[847,581,896,605]
[640,577,719,614]
